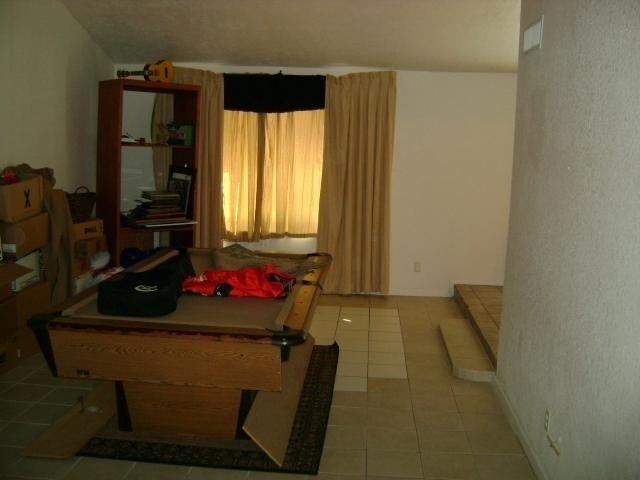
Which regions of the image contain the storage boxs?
[0,171,106,296]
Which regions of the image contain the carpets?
[76,341,339,474]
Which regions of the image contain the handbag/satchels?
[96,269,183,317]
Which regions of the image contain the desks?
[47,248,333,440]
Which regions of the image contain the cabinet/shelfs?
[96,78,202,271]
[0,283,47,374]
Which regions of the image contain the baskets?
[66,185,97,223]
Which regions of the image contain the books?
[121,190,186,226]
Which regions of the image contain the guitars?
[117,60,174,83]
